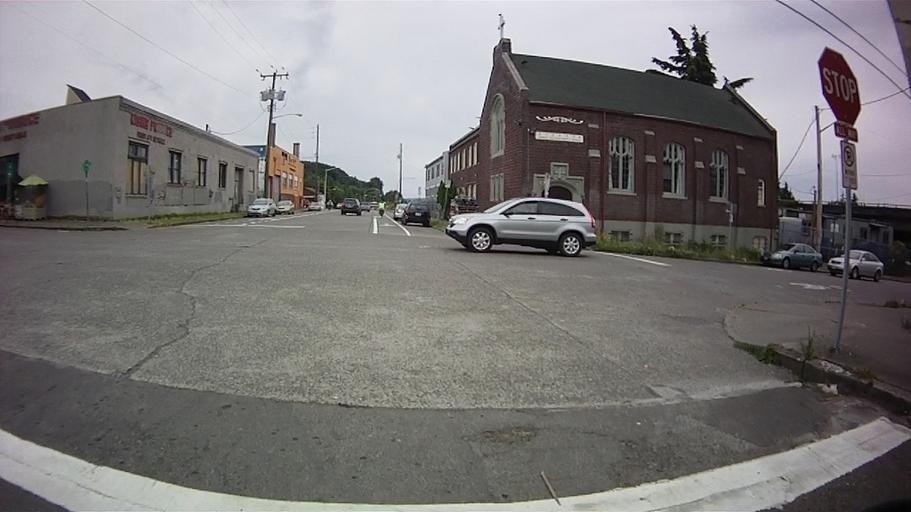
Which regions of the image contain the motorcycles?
[379,209,384,217]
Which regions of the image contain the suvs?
[402,202,431,227]
[445,197,597,257]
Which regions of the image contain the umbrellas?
[19,172,50,189]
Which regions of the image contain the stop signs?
[817,46,861,127]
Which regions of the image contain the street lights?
[264,114,301,198]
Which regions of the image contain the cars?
[308,202,323,211]
[828,249,884,282]
[336,198,378,216]
[394,203,409,221]
[764,243,823,272]
[246,198,295,218]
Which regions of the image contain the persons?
[378,199,386,214]
[24,184,48,209]
